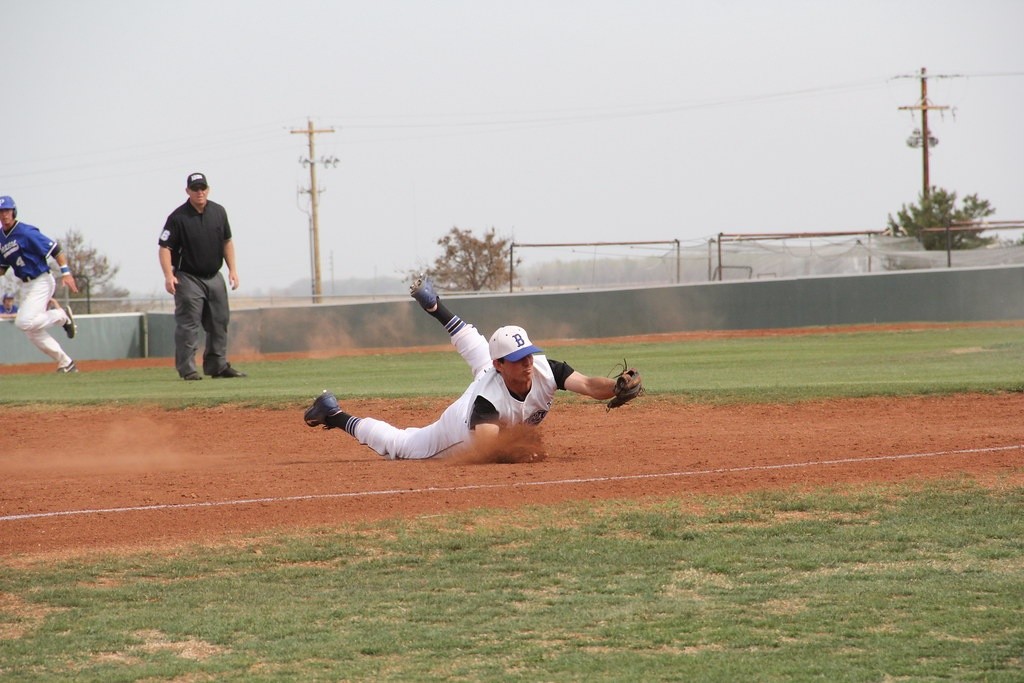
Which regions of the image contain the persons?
[0,195,79,373]
[304,274,643,460]
[159,173,245,380]
[0,294,18,318]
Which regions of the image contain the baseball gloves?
[606,368,644,410]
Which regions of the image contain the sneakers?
[63,306,76,338]
[57,360,76,374]
[304,390,343,430]
[212,361,246,378]
[409,273,438,309]
[184,371,202,379]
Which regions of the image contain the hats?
[187,173,208,186]
[489,325,543,362]
[3,292,14,299]
[0,196,16,209]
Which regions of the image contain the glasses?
[190,184,206,191]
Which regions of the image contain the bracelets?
[60,266,70,275]
